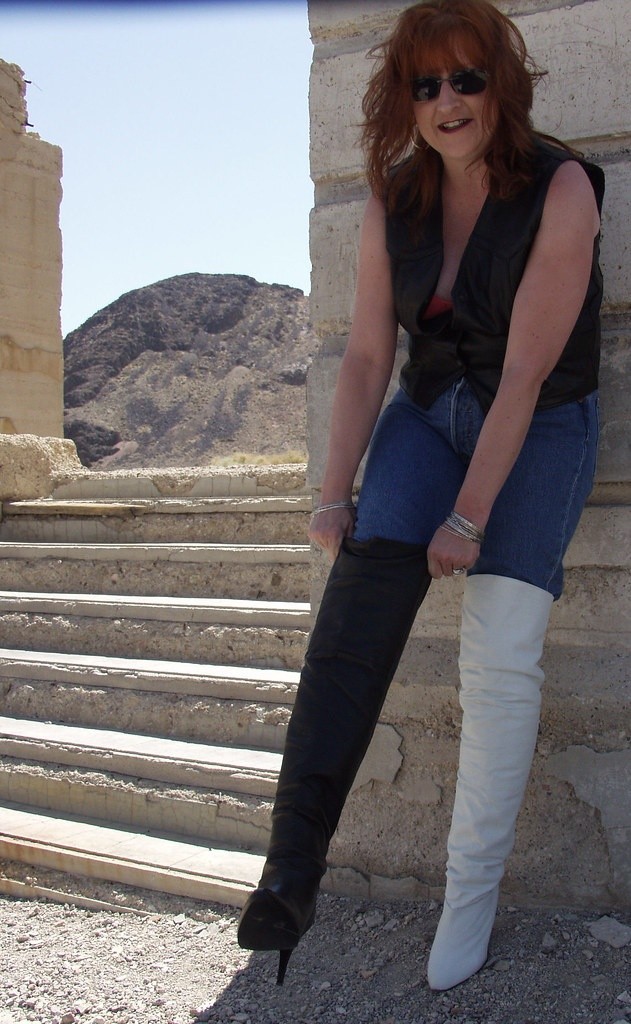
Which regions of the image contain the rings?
[453,569,463,574]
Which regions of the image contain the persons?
[237,0,606,991]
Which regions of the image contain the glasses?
[411,67,488,102]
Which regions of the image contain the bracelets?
[438,511,485,543]
[309,502,356,518]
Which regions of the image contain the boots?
[236,505,430,987]
[427,573,552,990]
